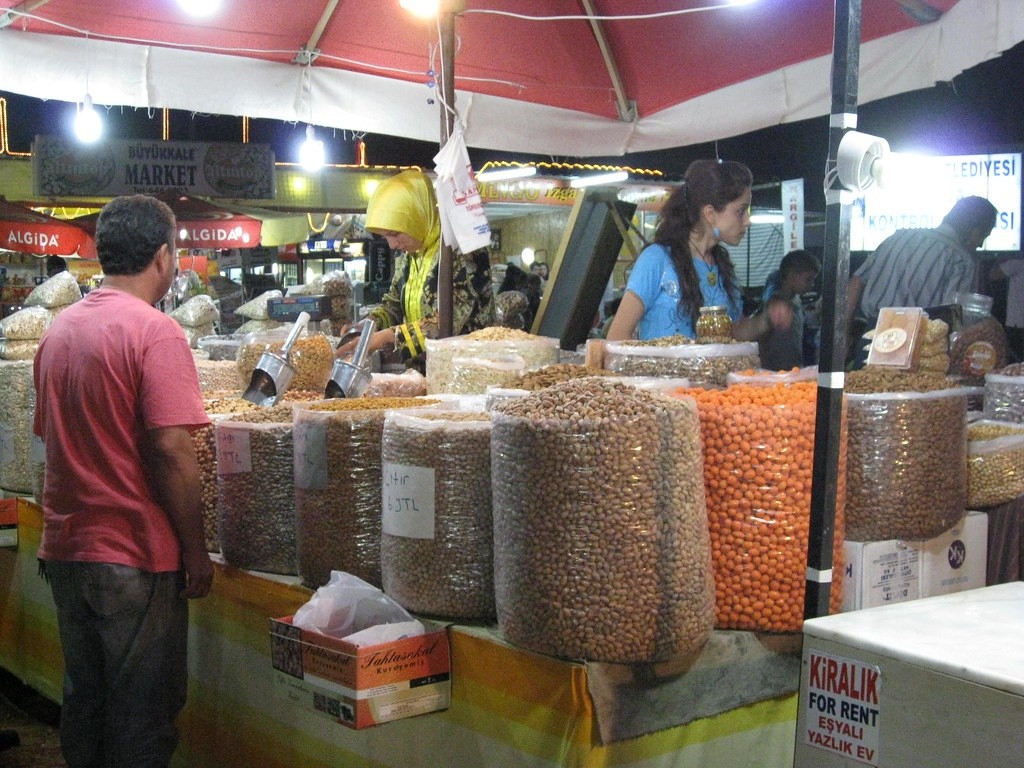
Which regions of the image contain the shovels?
[241,308,313,408]
[322,318,377,401]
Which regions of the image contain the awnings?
[71,194,260,248]
[207,199,310,246]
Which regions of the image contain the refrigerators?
[276,238,391,292]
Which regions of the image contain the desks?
[0,490,804,768]
[793,581,1024,768]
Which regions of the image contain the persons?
[845,195,996,371]
[47,257,66,278]
[756,250,820,370]
[608,160,793,345]
[32,194,215,768]
[988,252,1024,332]
[498,262,549,332]
[333,170,493,376]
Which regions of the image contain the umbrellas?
[0,196,98,260]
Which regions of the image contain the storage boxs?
[269,615,454,730]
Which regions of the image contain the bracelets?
[393,325,400,352]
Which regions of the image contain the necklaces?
[689,240,716,285]
[414,249,427,274]
